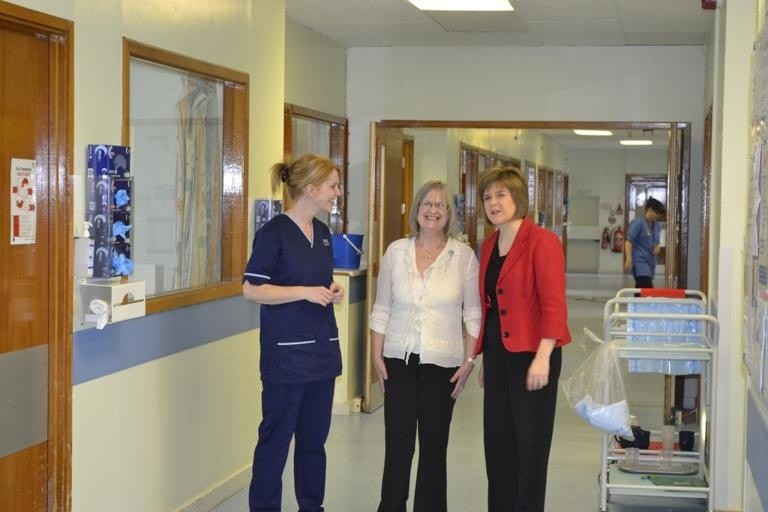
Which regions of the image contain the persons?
[469,166,571,511]
[240,152,345,512]
[367,181,483,511]
[624,198,665,296]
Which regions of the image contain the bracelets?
[463,352,474,368]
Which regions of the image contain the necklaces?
[416,237,443,258]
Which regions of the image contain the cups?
[656,451,672,473]
[678,431,694,450]
[661,425,674,451]
[624,448,639,469]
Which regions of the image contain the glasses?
[419,201,447,209]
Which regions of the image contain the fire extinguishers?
[609,226,625,252]
[602,227,609,249]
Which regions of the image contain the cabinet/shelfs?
[328,263,367,417]
